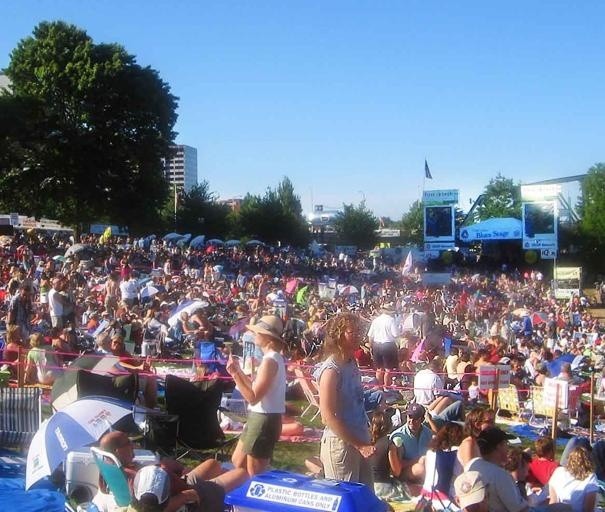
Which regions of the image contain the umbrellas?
[138,286,165,299]
[358,269,381,278]
[62,242,88,256]
[164,296,210,325]
[513,307,532,318]
[143,232,297,254]
[528,311,549,325]
[137,276,154,286]
[52,255,71,263]
[24,394,136,490]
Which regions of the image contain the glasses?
[477,419,494,425]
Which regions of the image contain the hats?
[401,403,426,418]
[381,304,396,315]
[478,427,517,449]
[454,471,489,510]
[133,465,170,505]
[245,316,290,346]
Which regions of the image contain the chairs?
[0,386,42,457]
[291,371,322,422]
[76,370,145,451]
[164,374,240,464]
[495,384,552,428]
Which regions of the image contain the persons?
[225,314,289,478]
[317,312,379,498]
[1,232,604,511]
